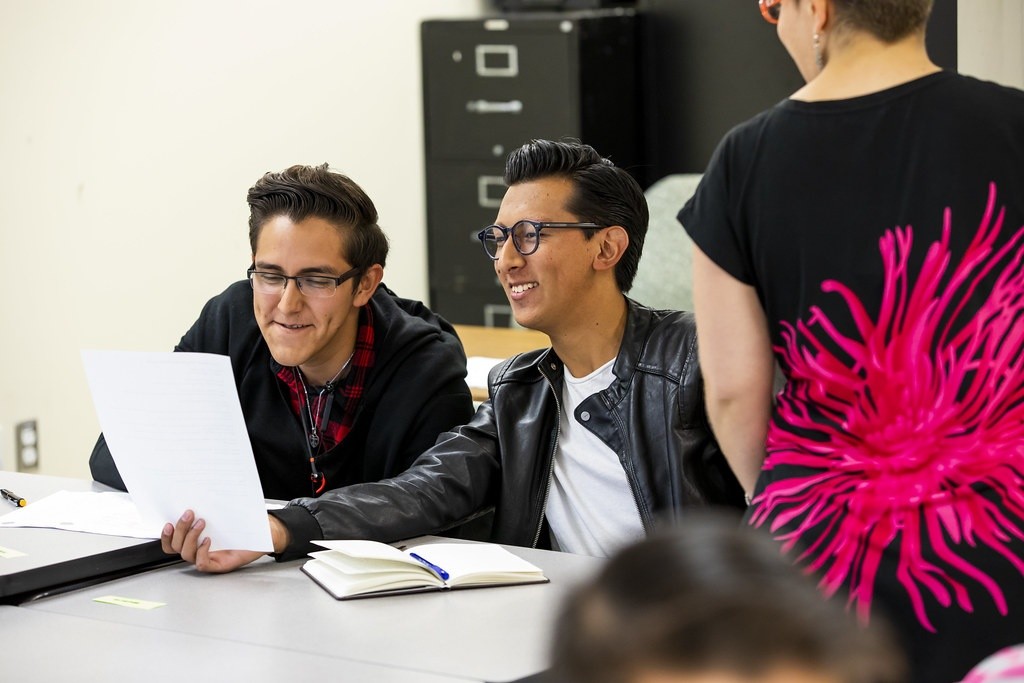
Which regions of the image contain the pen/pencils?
[410,551,450,580]
[0,488,26,507]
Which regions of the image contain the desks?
[0,470,609,683]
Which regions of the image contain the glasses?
[247,260,362,298]
[759,0,782,24]
[478,219,607,260]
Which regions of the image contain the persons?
[546,516,907,683]
[679,0,1024,682]
[163,138,746,569]
[92,166,495,541]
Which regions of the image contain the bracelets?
[745,492,756,506]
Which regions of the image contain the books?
[303,539,549,601]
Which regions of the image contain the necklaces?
[292,348,353,482]
